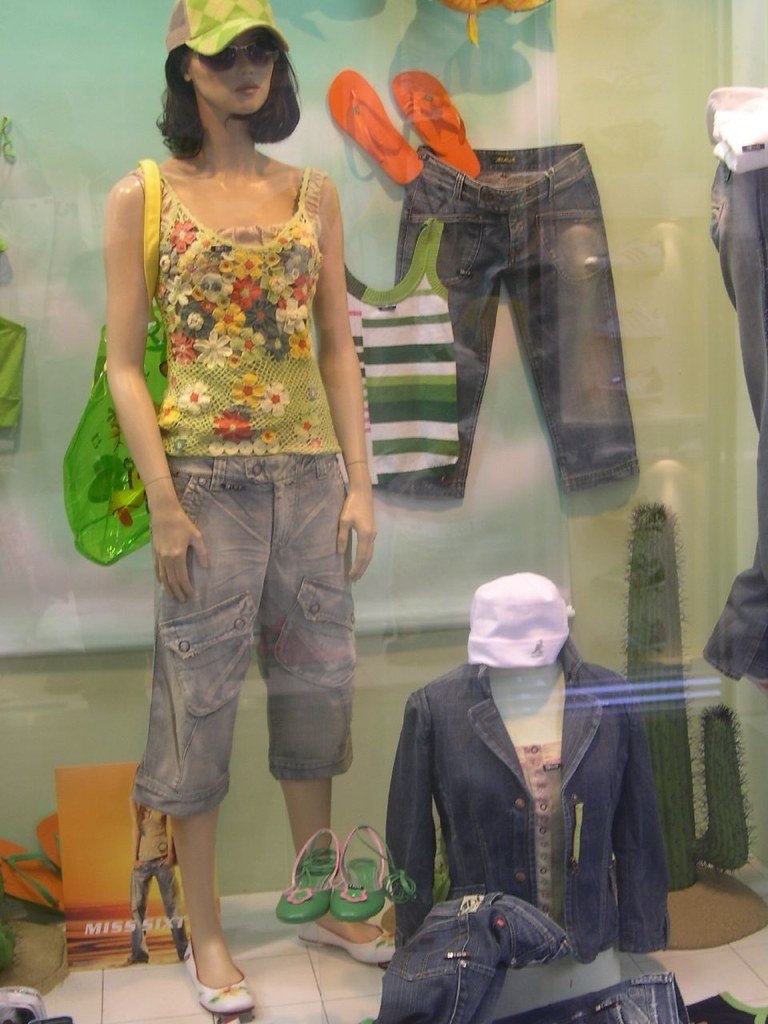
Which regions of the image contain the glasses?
[183,39,279,71]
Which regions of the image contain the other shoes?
[330,825,389,923]
[183,932,254,1015]
[300,910,396,963]
[274,828,339,925]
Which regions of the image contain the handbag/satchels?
[65,159,167,569]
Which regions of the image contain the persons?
[392,573,669,962]
[121,764,188,968]
[106,0,394,1014]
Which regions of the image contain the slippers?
[390,70,481,180]
[330,72,423,185]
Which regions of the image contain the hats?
[166,1,289,60]
[468,571,573,667]
